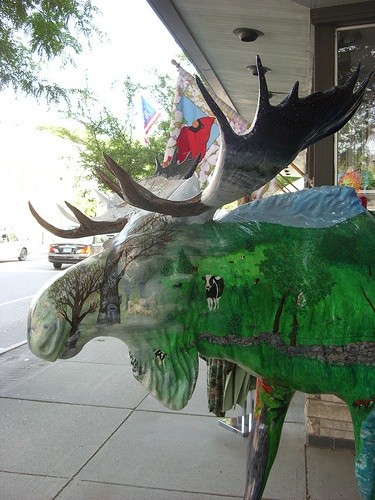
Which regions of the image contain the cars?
[0,230,29,262]
[48,226,109,268]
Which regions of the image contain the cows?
[202,274,224,311]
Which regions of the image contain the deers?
[26,52,375,500]
